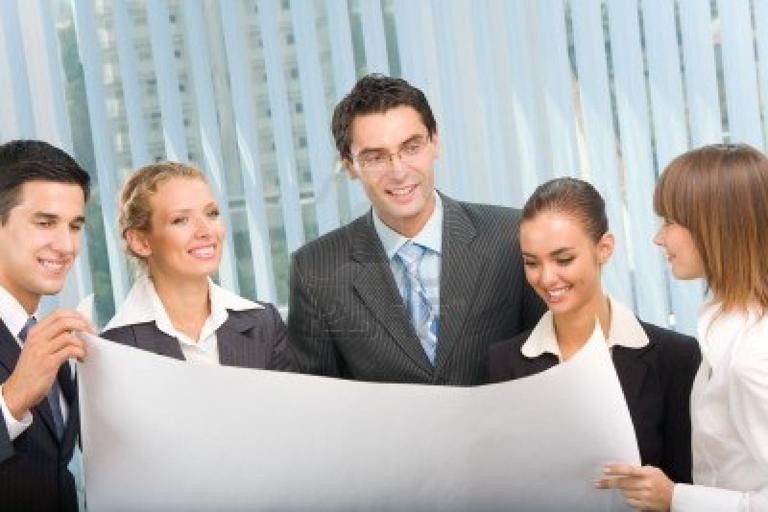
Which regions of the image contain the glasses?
[342,131,432,172]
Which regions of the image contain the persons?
[286,73,550,387]
[0,140,96,511]
[596,143,768,512]
[96,161,291,373]
[486,177,701,486]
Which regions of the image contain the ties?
[388,238,442,366]
[17,317,68,443]
